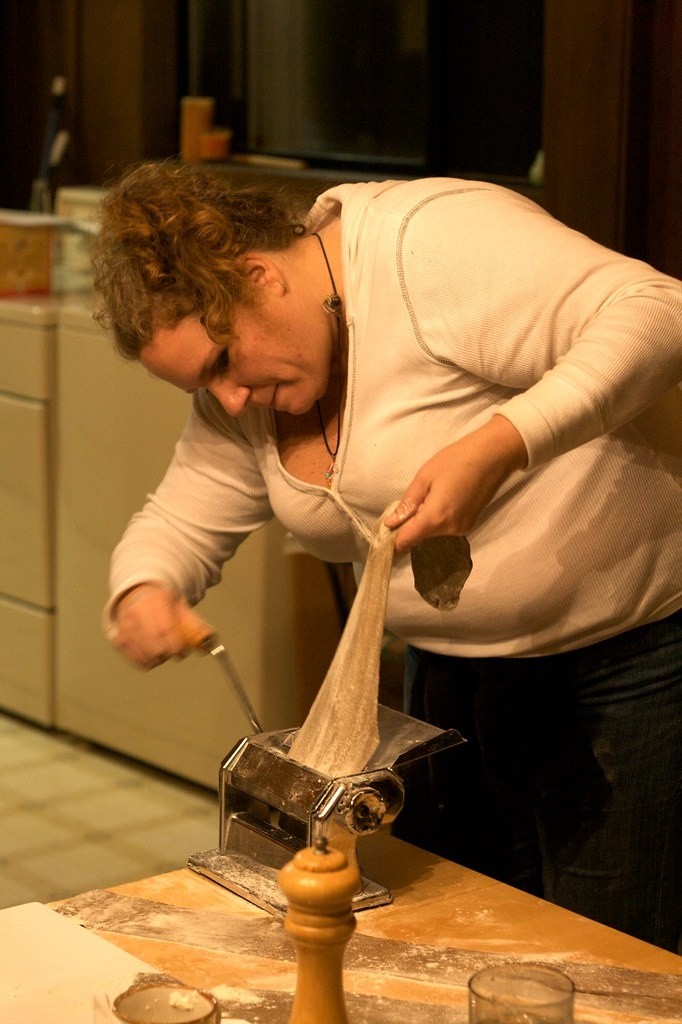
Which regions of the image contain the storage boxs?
[0,210,54,294]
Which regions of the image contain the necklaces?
[312,230,341,488]
[94,157,681,952]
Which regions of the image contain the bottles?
[200,131,229,159]
[277,837,360,1024]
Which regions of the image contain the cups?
[110,971,221,1024]
[181,97,212,164]
[1,211,60,295]
[467,961,576,1024]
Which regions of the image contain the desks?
[0,830,682,1024]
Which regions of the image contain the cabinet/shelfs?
[0,295,54,731]
[46,298,348,794]
